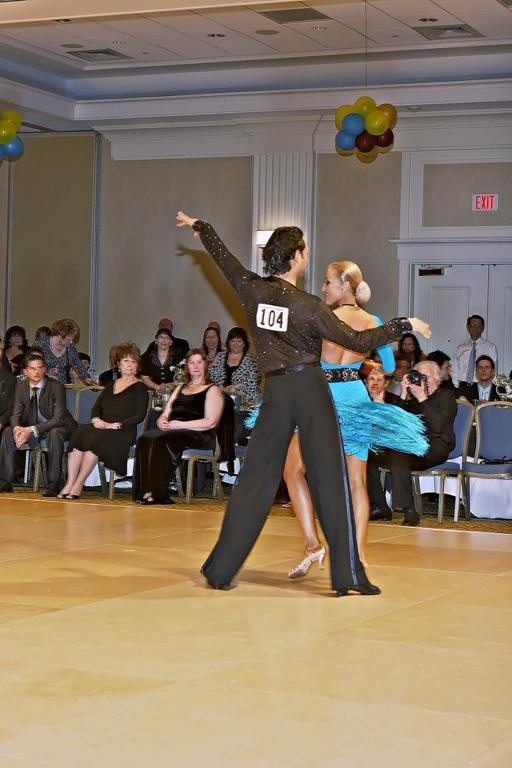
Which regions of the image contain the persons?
[1,319,260,504]
[281,260,398,580]
[364,315,500,525]
[175,209,433,597]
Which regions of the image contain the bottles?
[153,386,171,407]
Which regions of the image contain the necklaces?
[335,303,358,308]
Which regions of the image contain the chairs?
[453,400,512,523]
[98,390,153,501]
[174,390,234,504]
[39,385,106,493]
[19,382,87,484]
[212,445,249,498]
[411,399,475,524]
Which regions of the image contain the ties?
[481,390,487,401]
[466,342,476,383]
[29,387,39,426]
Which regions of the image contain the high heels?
[57,488,69,499]
[336,578,381,596]
[287,544,326,578]
[201,567,237,590]
[66,486,85,499]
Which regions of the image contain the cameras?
[407,369,427,387]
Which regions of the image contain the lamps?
[255,229,278,249]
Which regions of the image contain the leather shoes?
[369,505,393,521]
[0,482,12,493]
[41,487,60,497]
[401,510,419,526]
[136,495,156,505]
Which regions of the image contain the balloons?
[0,107,23,164]
[332,94,399,162]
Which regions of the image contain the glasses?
[468,323,482,328]
[27,366,44,371]
